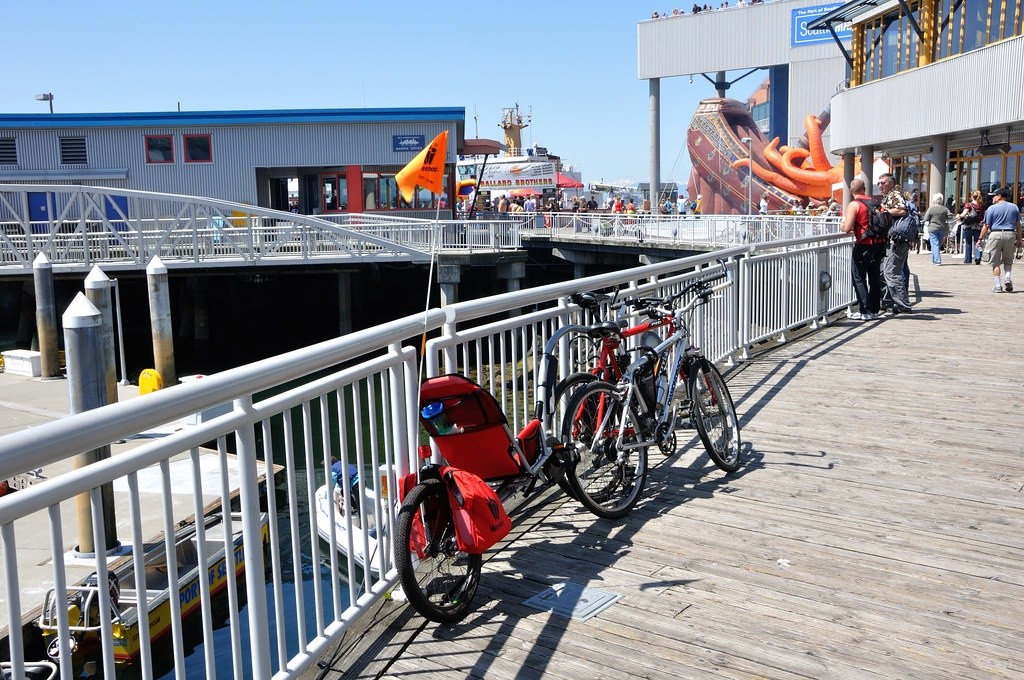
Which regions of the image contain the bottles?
[978,245,983,252]
[654,369,669,409]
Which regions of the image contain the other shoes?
[993,285,1002,292]
[964,260,972,264]
[1005,277,1013,291]
[974,261,980,265]
[886,304,911,313]
[931,262,941,266]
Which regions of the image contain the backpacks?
[430,464,512,555]
[757,203,763,210]
[690,200,697,211]
[854,198,892,247]
[884,189,918,243]
[399,468,431,560]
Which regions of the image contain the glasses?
[878,182,886,185]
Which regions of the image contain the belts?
[992,229,1015,231]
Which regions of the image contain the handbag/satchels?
[963,203,978,225]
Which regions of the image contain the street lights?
[741,137,753,216]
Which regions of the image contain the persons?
[382,194,702,229]
[759,172,984,321]
[321,456,366,529]
[652,0,762,19]
[975,188,1022,293]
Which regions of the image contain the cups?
[422,402,451,435]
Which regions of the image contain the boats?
[37,510,270,677]
[314,483,399,579]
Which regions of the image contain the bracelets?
[978,238,982,240]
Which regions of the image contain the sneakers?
[847,311,879,321]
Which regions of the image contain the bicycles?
[393,272,743,624]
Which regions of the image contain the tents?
[555,172,584,197]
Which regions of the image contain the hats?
[680,195,683,198]
[988,188,1008,197]
[321,456,336,465]
[828,197,837,202]
[808,201,814,206]
[697,194,703,200]
[580,196,586,199]
